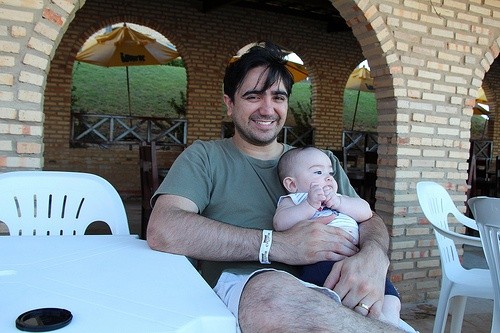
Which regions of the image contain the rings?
[357,303,371,312]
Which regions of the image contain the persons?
[146,39,420,333]
[274,146,401,327]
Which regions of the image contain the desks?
[0,235,237,333]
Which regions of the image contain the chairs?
[0,172,131,236]
[468,196,500,333]
[343,148,500,212]
[416,181,500,333]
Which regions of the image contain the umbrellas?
[230,55,309,85]
[75,22,181,136]
[472,93,491,115]
[345,65,377,134]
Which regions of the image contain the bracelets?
[258,228,273,264]
